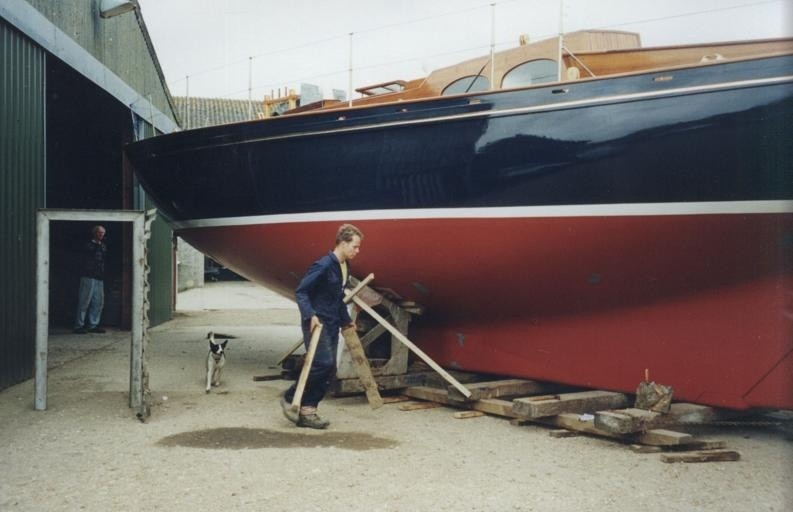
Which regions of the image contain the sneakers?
[72,327,88,334]
[280,398,300,424]
[292,408,330,429]
[88,327,106,333]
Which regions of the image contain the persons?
[73,226,110,334]
[280,223,362,429]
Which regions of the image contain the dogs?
[205,329,229,394]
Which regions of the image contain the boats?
[123,29,792,413]
